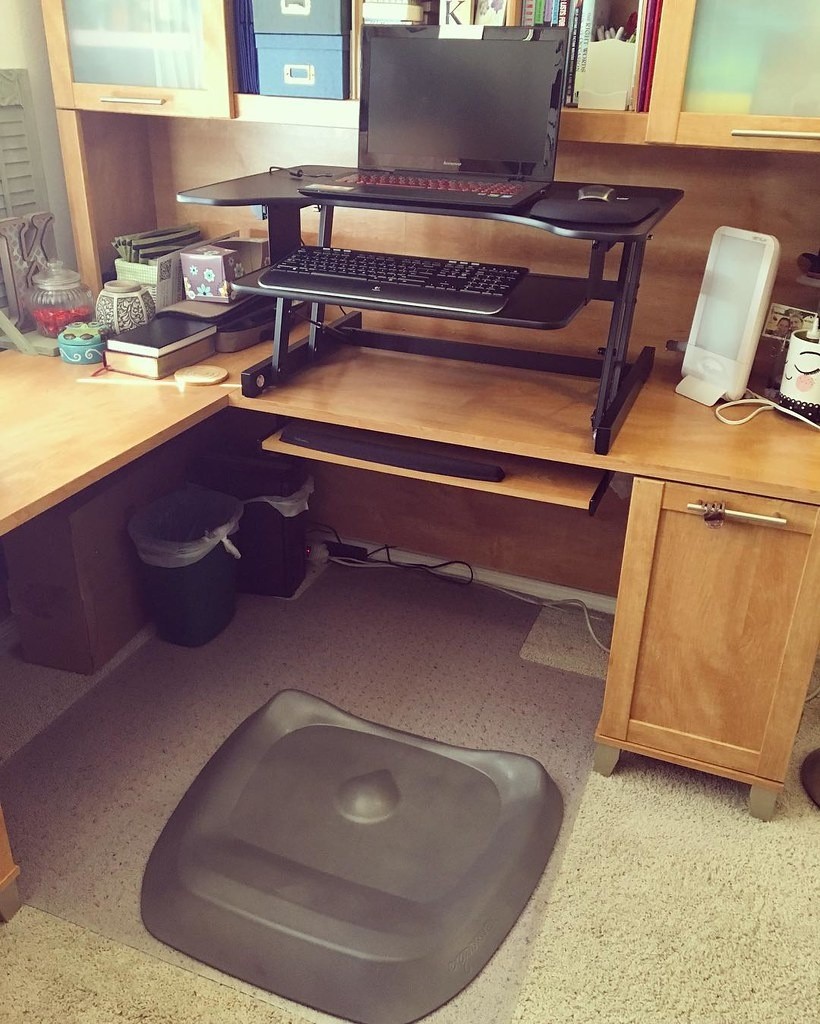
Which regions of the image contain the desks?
[0,320,820,821]
[176,167,685,456]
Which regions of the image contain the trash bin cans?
[127,485,245,650]
[214,462,314,598]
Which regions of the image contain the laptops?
[298,24,569,212]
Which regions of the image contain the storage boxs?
[252,0,350,99]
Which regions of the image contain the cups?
[776,329,820,425]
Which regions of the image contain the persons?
[772,315,803,339]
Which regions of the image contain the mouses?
[577,185,617,202]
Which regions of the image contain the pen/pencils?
[596,12,637,43]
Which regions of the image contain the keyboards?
[257,246,529,314]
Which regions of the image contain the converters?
[324,541,368,564]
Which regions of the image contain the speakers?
[674,225,781,407]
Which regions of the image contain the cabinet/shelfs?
[40,0,820,312]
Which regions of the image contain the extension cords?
[305,542,329,563]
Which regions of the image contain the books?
[349,0,362,100]
[103,318,216,380]
[563,0,665,112]
[363,0,568,26]
